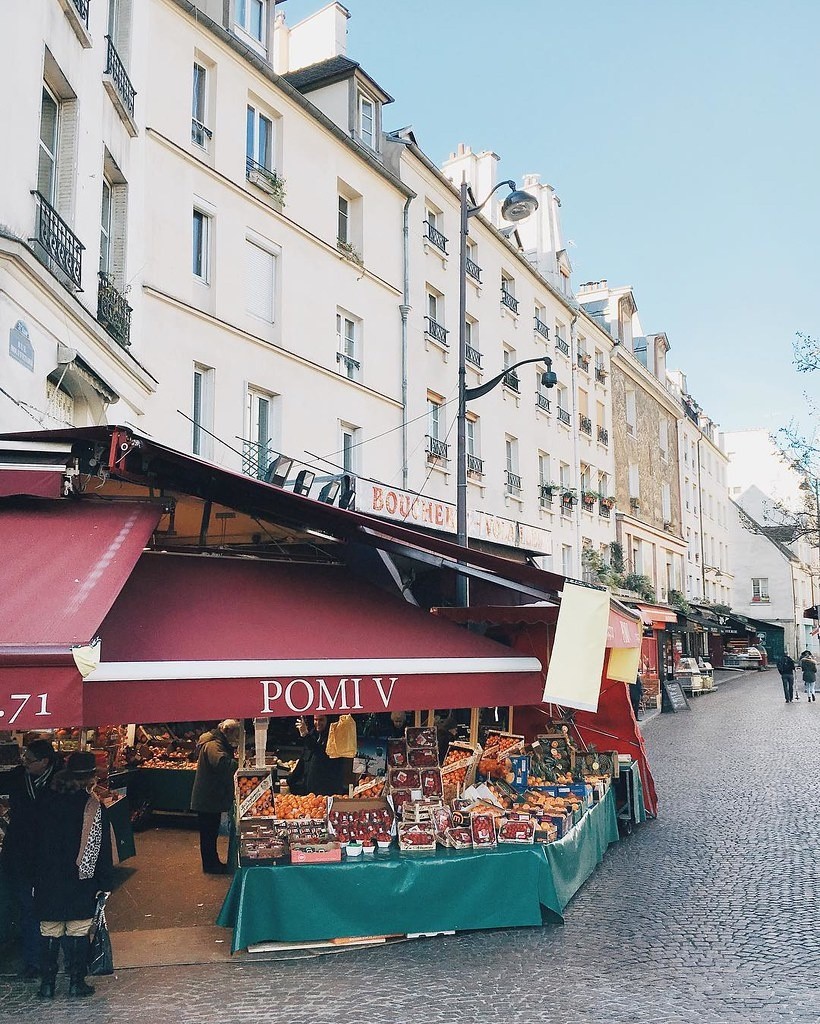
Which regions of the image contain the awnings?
[675,611,725,634]
[637,605,677,623]
[730,616,756,633]
[0,425,645,730]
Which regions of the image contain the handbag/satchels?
[88,893,114,976]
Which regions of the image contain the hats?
[67,752,96,772]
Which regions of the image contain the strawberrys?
[389,729,441,805]
[402,817,534,845]
[329,809,393,847]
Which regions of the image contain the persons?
[379,711,409,738]
[800,652,817,702]
[629,674,644,722]
[190,720,251,874]
[777,651,796,703]
[0,739,113,997]
[295,715,344,797]
[437,717,458,766]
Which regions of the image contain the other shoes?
[636,719,642,721]
[203,862,227,874]
[812,694,815,701]
[808,697,810,702]
[786,701,789,703]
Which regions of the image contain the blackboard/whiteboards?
[663,679,691,710]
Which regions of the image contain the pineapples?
[540,740,610,775]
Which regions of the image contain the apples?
[486,736,521,752]
[441,750,473,785]
[238,777,274,816]
[275,792,350,820]
[468,772,603,819]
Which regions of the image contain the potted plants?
[543,483,616,512]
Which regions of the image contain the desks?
[217,818,541,957]
[535,784,619,924]
[97,792,138,866]
[137,766,231,829]
[618,759,646,835]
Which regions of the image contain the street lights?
[458,181,558,630]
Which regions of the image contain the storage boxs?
[88,722,632,864]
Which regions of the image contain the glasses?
[23,754,41,764]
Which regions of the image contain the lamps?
[338,489,356,510]
[263,455,294,490]
[318,480,341,504]
[293,470,316,496]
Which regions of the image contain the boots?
[37,936,61,999]
[61,936,95,997]
